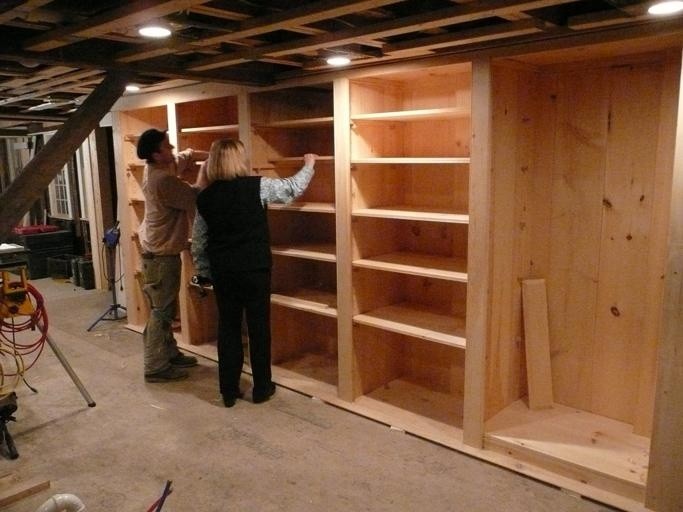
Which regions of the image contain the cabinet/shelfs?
[340,51,539,447]
[462,16,683,512]
[109,86,185,352]
[170,82,252,383]
[238,72,344,408]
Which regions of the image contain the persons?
[191,138,318,406]
[135,128,210,382]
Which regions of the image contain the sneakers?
[221,387,240,408]
[144,352,198,382]
[253,383,275,404]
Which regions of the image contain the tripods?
[87,247,129,332]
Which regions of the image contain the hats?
[137,128,167,159]
